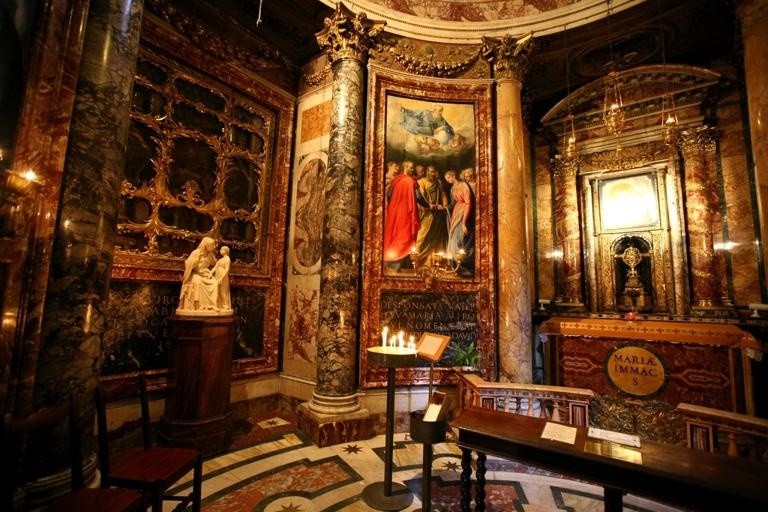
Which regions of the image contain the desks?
[450,404,766,512]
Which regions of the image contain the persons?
[384,105,475,279]
[178,237,233,311]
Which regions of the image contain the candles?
[365,323,421,358]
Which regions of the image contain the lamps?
[0,165,44,215]
[405,240,469,292]
[557,1,683,178]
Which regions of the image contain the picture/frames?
[378,86,483,285]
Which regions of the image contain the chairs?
[1,387,153,512]
[90,371,202,512]
